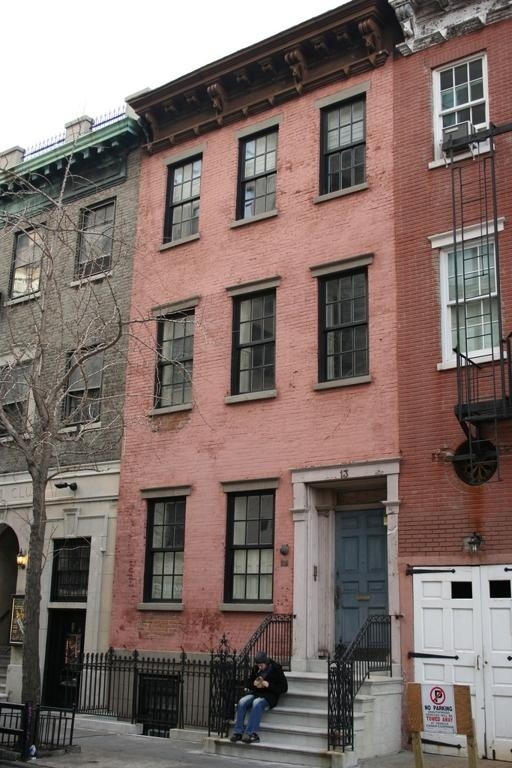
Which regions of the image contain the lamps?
[16,547,25,569]
[54,483,77,491]
[467,531,481,553]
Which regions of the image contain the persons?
[228,650,288,746]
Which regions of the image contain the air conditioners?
[441,121,478,154]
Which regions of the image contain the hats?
[254,652,266,663]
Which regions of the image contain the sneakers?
[231,732,259,742]
